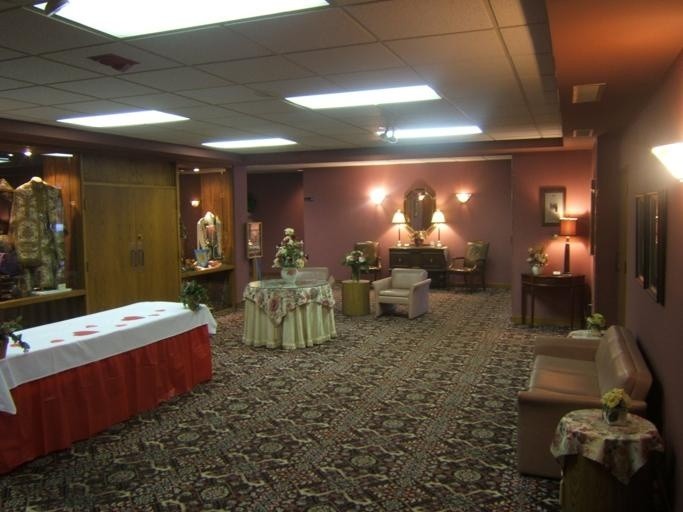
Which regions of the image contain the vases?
[531,264,539,275]
[590,326,602,336]
[602,405,628,425]
[279,267,300,282]
[352,264,359,281]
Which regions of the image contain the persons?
[196,211,223,260]
[6,176,66,290]
[0,178,14,250]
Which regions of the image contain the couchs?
[517,325,654,481]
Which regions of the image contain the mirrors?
[179,168,202,265]
[0,153,44,253]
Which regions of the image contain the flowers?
[585,312,604,328]
[341,250,366,281]
[598,387,633,421]
[526,247,548,268]
[270,228,308,269]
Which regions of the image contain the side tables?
[566,328,607,340]
[340,279,370,317]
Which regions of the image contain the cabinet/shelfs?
[388,245,449,288]
[75,182,182,315]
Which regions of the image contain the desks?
[0,288,87,309]
[0,301,217,476]
[520,272,586,330]
[241,278,338,350]
[181,263,235,313]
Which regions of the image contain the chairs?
[299,267,335,289]
[448,240,490,293]
[354,240,383,280]
[371,267,432,320]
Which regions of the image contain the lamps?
[456,193,471,203]
[559,218,577,274]
[391,208,406,246]
[378,109,396,144]
[430,207,446,247]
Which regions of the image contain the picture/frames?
[635,193,648,290]
[645,188,664,304]
[541,187,566,226]
[246,221,263,259]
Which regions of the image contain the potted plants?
[177,279,213,311]
[0,316,29,359]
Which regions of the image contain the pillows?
[594,324,637,398]
